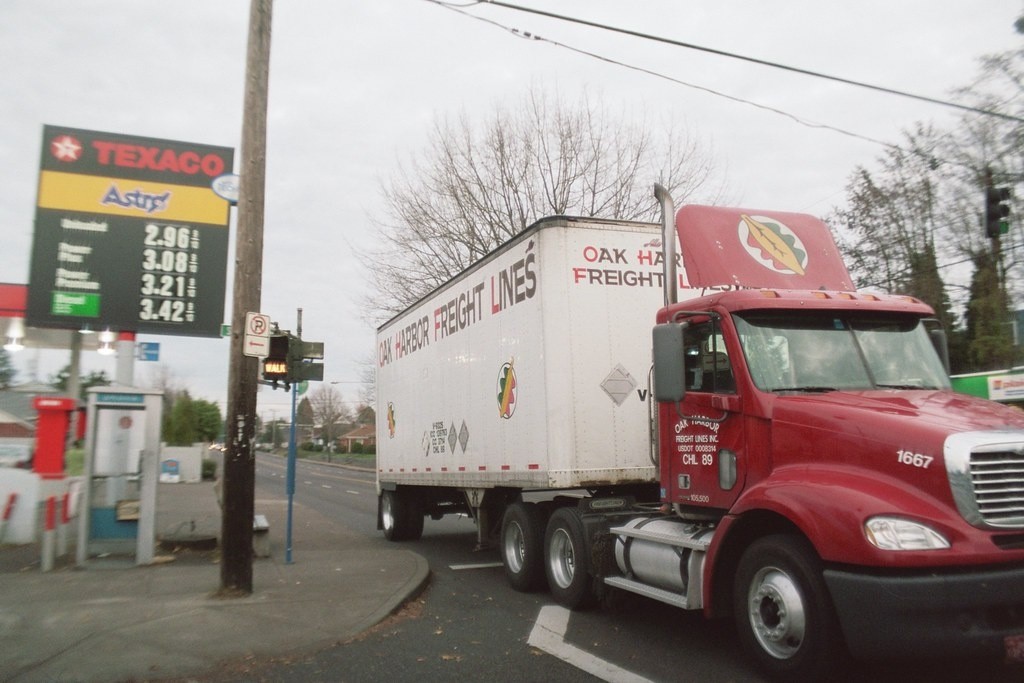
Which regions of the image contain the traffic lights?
[262,331,292,381]
[985,185,1011,239]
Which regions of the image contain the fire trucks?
[373,182,1024,683]
